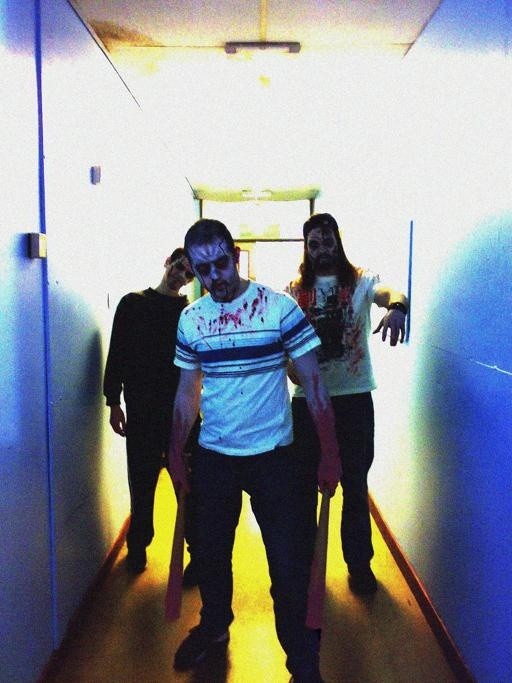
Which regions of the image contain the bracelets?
[386,300,409,316]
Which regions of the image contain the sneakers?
[349,568,377,595]
[183,561,198,586]
[173,623,230,670]
[126,550,146,573]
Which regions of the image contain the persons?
[280,210,415,595]
[163,217,342,681]
[99,243,211,589]
